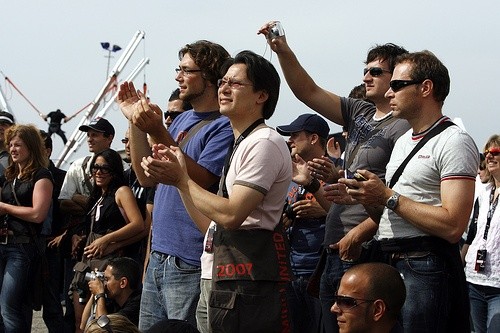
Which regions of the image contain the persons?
[0,20,500,333]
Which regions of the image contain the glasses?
[92,163,110,174]
[121,137,129,143]
[483,148,500,158]
[175,68,202,75]
[86,314,113,333]
[335,291,376,309]
[364,67,390,76]
[389,80,423,92]
[217,79,253,89]
[164,110,183,121]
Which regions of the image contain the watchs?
[384,192,402,210]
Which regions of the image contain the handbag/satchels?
[208,227,296,333]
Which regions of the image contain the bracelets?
[93,291,107,300]
[302,177,320,194]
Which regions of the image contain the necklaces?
[129,168,139,189]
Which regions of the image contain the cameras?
[267,20,285,39]
[285,193,305,226]
[85,267,105,283]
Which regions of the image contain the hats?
[276,113,330,136]
[0,111,14,124]
[79,117,115,137]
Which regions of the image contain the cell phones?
[344,169,356,189]
[474,249,486,271]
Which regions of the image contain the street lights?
[100,41,123,108]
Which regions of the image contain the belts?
[377,238,460,252]
